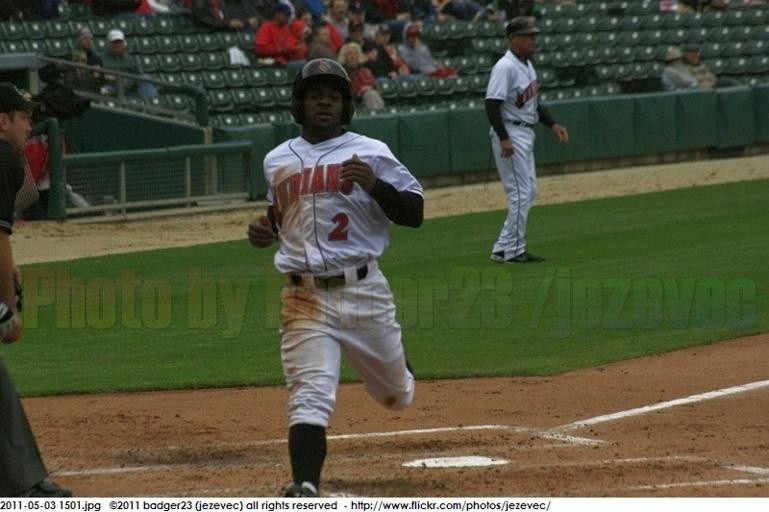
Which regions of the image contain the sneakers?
[490,250,545,264]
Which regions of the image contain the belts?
[291,266,367,289]
[514,122,534,127]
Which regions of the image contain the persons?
[0,82,72,497]
[662,45,698,93]
[685,41,736,90]
[247,57,424,498]
[484,16,569,263]
[1,0,460,110]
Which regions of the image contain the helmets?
[292,57,354,125]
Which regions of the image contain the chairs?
[0,1,768,128]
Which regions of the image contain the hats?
[267,3,291,16]
[406,25,419,36]
[0,81,42,110]
[506,18,540,37]
[348,18,365,32]
[373,24,392,37]
[73,26,93,46]
[107,29,124,43]
